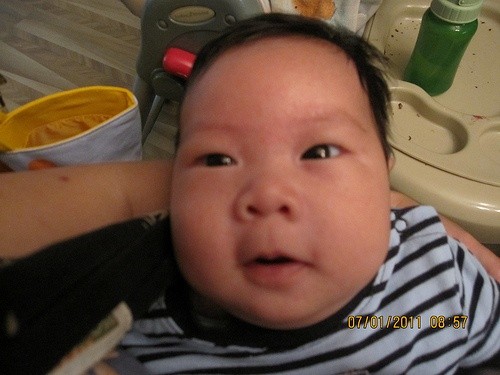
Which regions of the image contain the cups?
[403,0,483,96]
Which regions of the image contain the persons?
[0,160,500,283]
[0,14,500,374]
